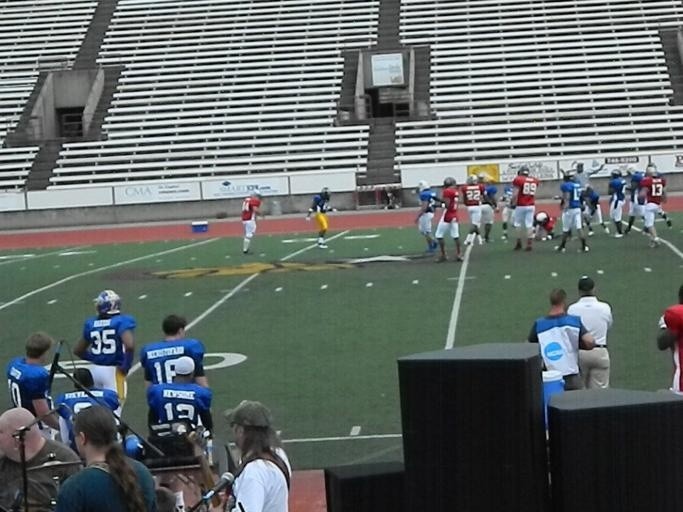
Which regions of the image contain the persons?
[415,163,672,261]
[1,291,291,511]
[241,189,260,253]
[657,284,683,395]
[306,187,337,248]
[528,277,613,391]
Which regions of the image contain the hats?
[222,399,274,428]
[578,276,596,292]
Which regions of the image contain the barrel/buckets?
[271,201,282,216]
[543,371,567,441]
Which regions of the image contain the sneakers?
[456,254,464,262]
[463,234,509,246]
[613,219,673,248]
[577,245,590,253]
[525,247,532,251]
[604,225,610,235]
[514,243,522,250]
[425,240,438,253]
[434,254,449,263]
[554,245,566,254]
[243,250,254,255]
[588,231,595,236]
[318,242,329,249]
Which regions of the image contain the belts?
[594,344,609,348]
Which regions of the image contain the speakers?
[547,386,683,511]
[323,461,407,512]
[397,342,547,510]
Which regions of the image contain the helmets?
[417,162,658,191]
[320,186,332,196]
[92,289,122,315]
[250,190,262,197]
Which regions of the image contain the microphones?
[48,341,61,372]
[205,472,236,500]
